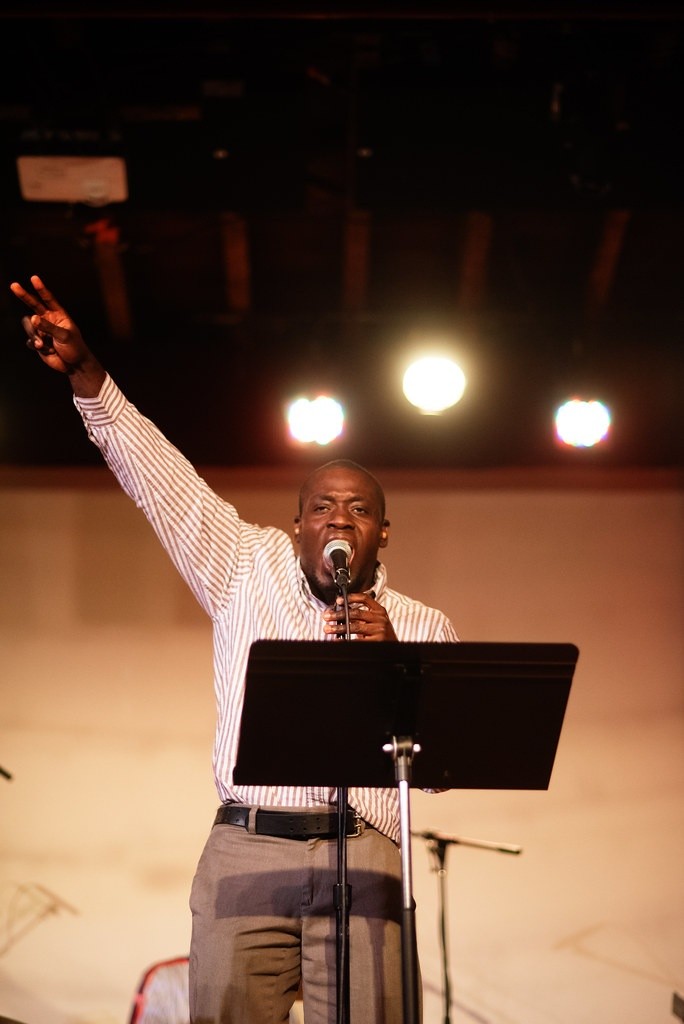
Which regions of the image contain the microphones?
[324,539,353,587]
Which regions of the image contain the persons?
[10,276,462,1024]
[131,957,190,1024]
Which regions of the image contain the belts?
[212,806,374,838]
